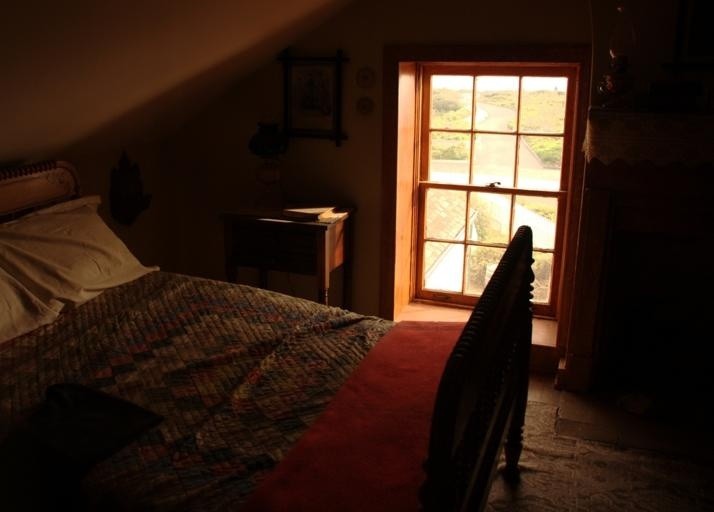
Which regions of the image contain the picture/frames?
[277,49,348,147]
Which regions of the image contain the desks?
[224,206,355,307]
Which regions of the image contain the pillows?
[0,195,158,342]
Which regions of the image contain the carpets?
[483,430,714,512]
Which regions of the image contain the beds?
[0,160,533,512]
